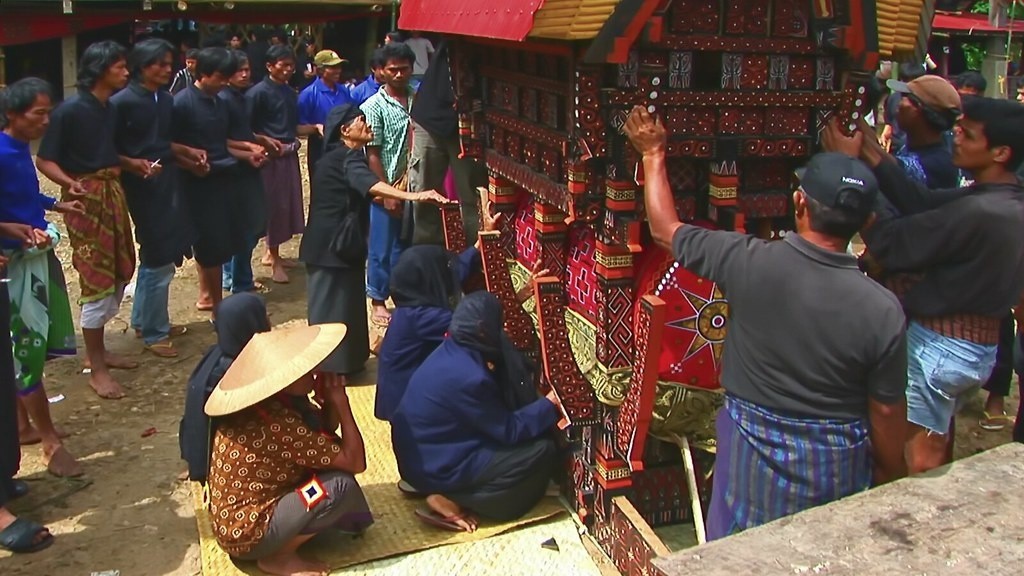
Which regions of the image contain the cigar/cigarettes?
[151,159,161,168]
[1,279,12,283]
[145,174,148,179]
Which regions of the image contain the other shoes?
[253,283,271,294]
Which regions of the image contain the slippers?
[145,341,177,356]
[0,517,53,552]
[979,410,1007,431]
[170,324,187,336]
[11,480,28,496]
[370,301,392,327]
[415,508,470,532]
[398,479,424,495]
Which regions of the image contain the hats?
[886,74,962,119]
[794,151,878,216]
[338,106,364,126]
[204,323,348,415]
[313,49,347,68]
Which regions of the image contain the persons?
[622,104,909,541]
[822,61,1024,479]
[0,23,567,576]
